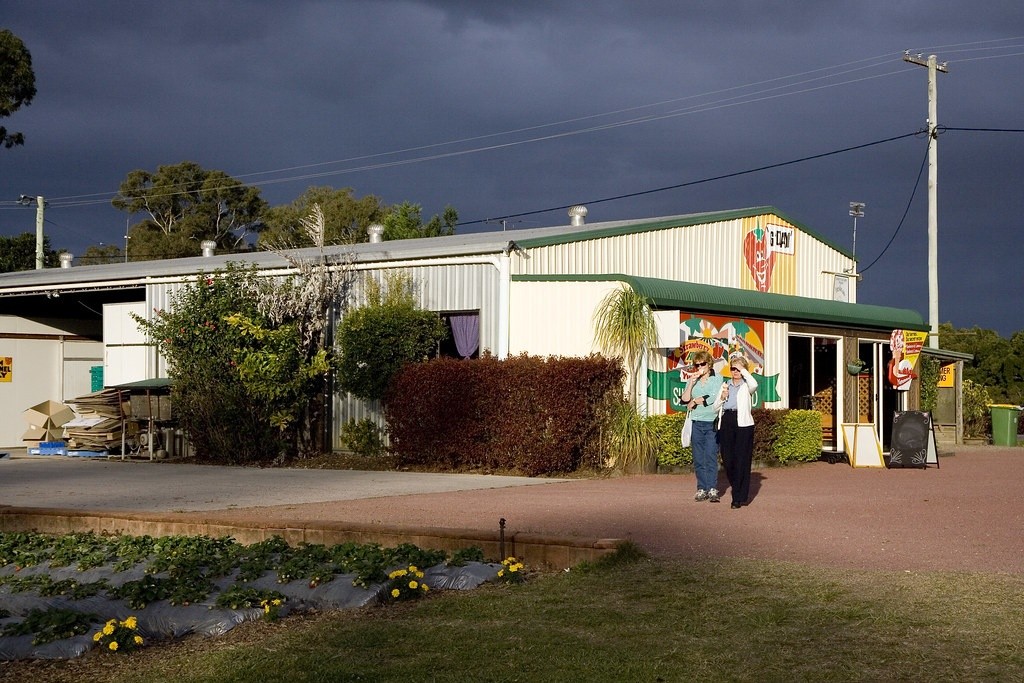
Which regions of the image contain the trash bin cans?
[987,403,1024,448]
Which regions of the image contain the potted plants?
[847,359,866,374]
[592,282,667,476]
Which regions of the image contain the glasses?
[730,367,740,371]
[695,361,708,367]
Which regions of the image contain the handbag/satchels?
[681,409,692,449]
[713,415,720,432]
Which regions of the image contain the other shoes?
[729,500,742,509]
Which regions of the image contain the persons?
[713,357,758,509]
[681,351,724,500]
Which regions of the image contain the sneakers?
[694,489,709,501]
[708,487,721,502]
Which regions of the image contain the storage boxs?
[39,442,66,448]
[22,428,70,447]
[22,399,76,431]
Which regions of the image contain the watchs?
[693,398,697,405]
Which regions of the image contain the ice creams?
[693,370,703,381]
[890,330,904,377]
[722,384,728,400]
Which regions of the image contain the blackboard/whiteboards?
[888,409,930,470]
